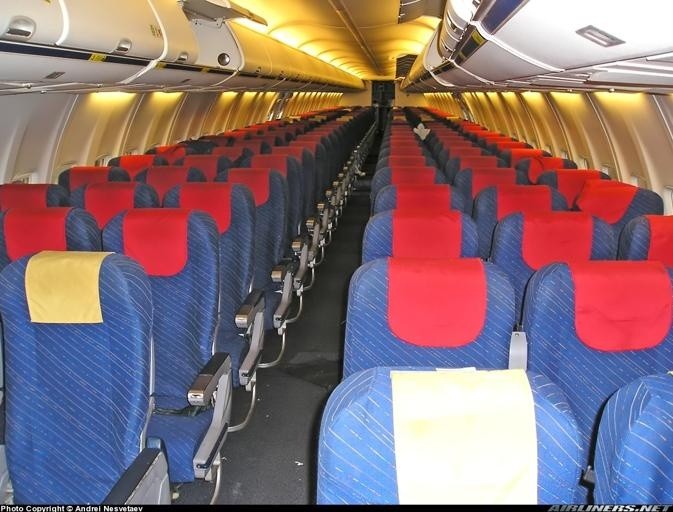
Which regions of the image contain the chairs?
[0,104,673,504]
